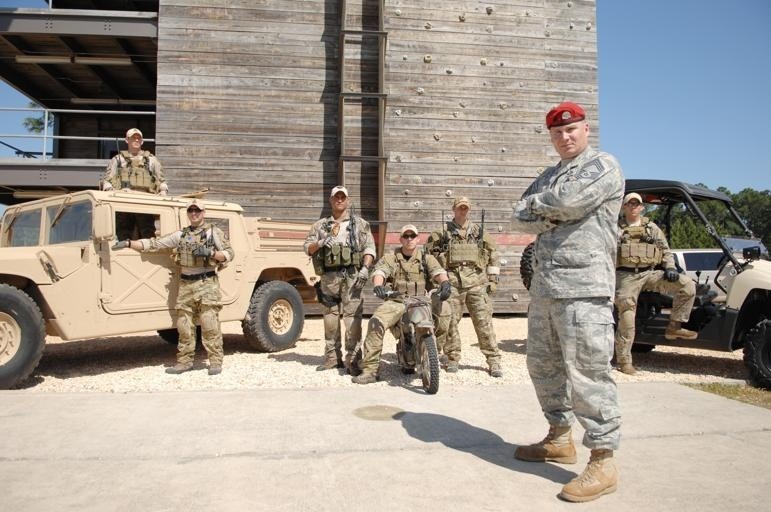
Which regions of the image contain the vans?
[664,247,733,301]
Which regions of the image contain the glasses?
[187,208,202,212]
[403,234,417,238]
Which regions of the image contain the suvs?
[519,178,771,391]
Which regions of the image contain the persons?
[425,194,505,378]
[303,185,378,377]
[508,99,624,504]
[99,127,170,240]
[612,191,702,378]
[110,197,234,375]
[351,223,453,386]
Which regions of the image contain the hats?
[331,185,348,197]
[453,196,470,209]
[546,101,585,131]
[400,224,418,237]
[126,128,143,138]
[186,199,204,211]
[623,192,642,205]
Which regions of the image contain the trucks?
[0,184,322,389]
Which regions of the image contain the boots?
[618,362,636,374]
[559,447,619,503]
[515,425,578,464]
[664,321,697,340]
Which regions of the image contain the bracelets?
[125,238,130,248]
[362,263,370,270]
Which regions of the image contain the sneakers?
[489,363,503,377]
[165,363,193,374]
[316,358,344,371]
[351,372,377,383]
[346,361,362,376]
[208,365,223,375]
[446,361,458,372]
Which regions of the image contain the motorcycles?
[374,286,450,396]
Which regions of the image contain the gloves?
[111,240,127,250]
[486,281,497,297]
[433,281,451,301]
[318,237,334,248]
[351,266,369,290]
[372,285,388,299]
[192,247,211,257]
[663,268,679,282]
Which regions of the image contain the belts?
[615,266,650,273]
[323,264,355,273]
[180,271,216,281]
